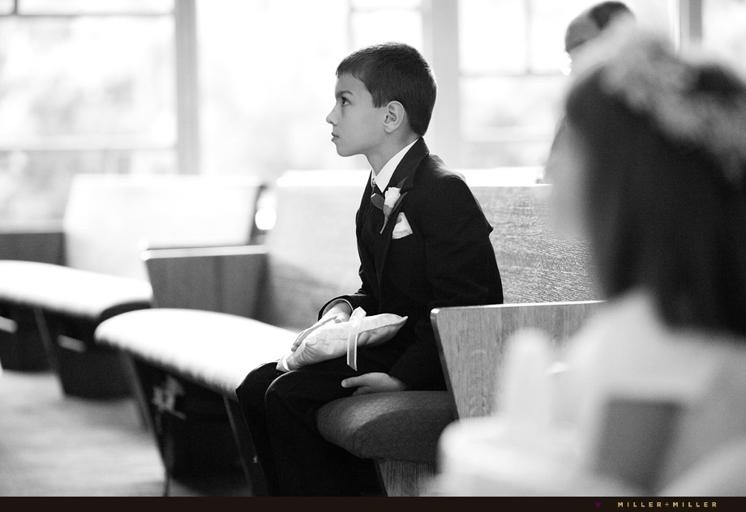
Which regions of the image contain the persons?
[544,0,635,183]
[420,13,745,497]
[235,43,504,496]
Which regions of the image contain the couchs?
[0,171,263,399]
[93,173,610,499]
